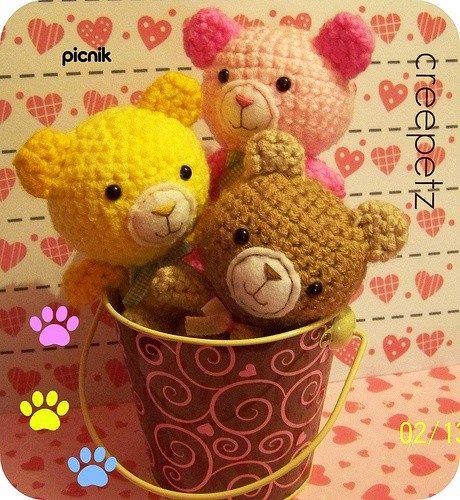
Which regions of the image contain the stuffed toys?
[12,70,212,335]
[147,128,411,339]
[180,6,377,274]
